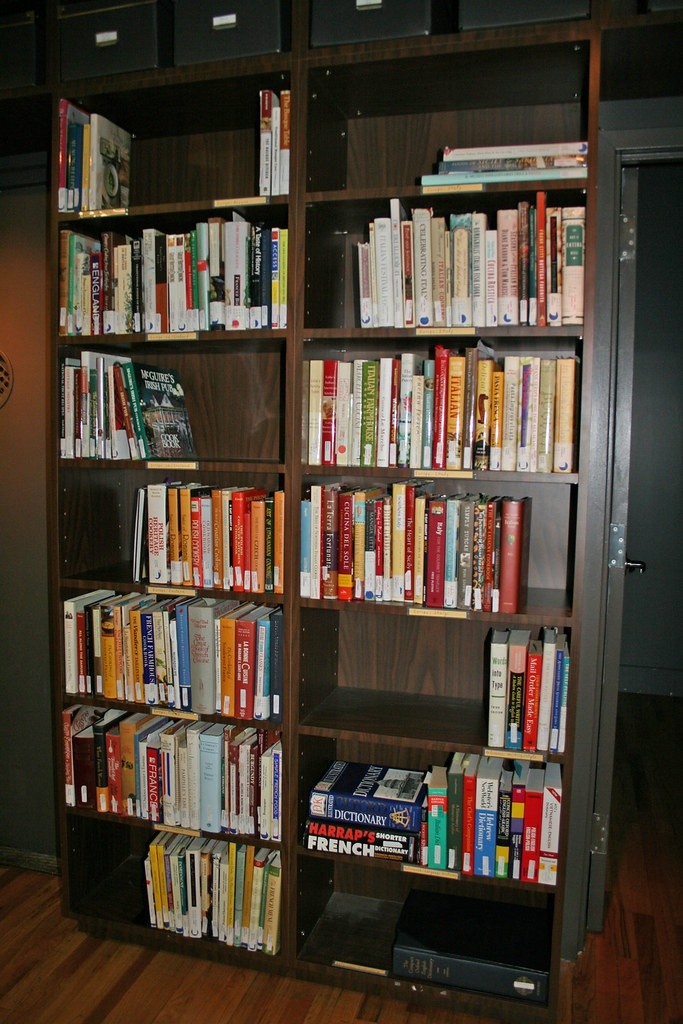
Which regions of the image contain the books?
[57,216,288,330]
[58,90,290,212]
[301,479,533,614]
[144,831,283,956]
[390,889,550,1005]
[61,705,283,843]
[133,479,284,593]
[488,626,570,753]
[59,351,198,461]
[421,142,589,185]
[357,191,586,327]
[64,590,283,725]
[303,752,562,885]
[303,341,582,473]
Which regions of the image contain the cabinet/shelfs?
[282,16,604,1007]
[47,50,305,987]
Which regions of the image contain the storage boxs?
[56,0,160,82]
[311,0,431,48]
[0,19,36,92]
[459,0,591,33]
[174,0,282,66]
